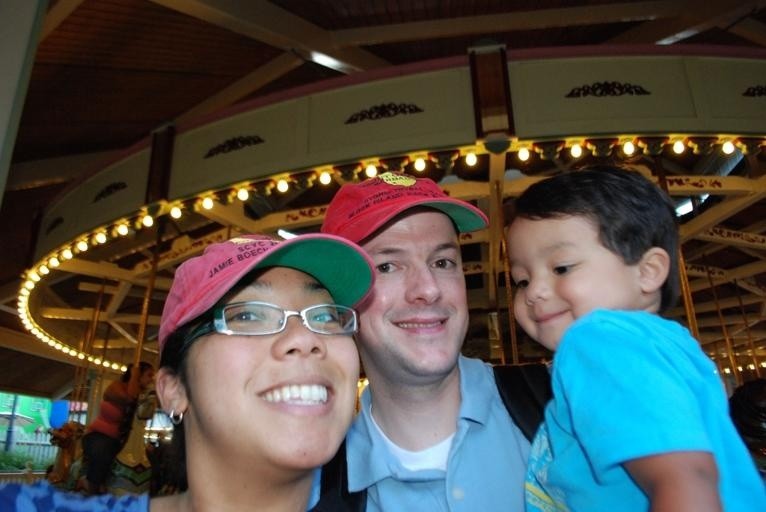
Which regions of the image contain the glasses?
[173,298,358,361]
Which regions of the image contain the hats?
[318,173,492,247]
[155,232,376,351]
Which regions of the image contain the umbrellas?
[0,411,35,428]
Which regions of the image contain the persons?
[309,170,553,511]
[0,234,376,512]
[507,165,766,512]
[82,361,155,495]
